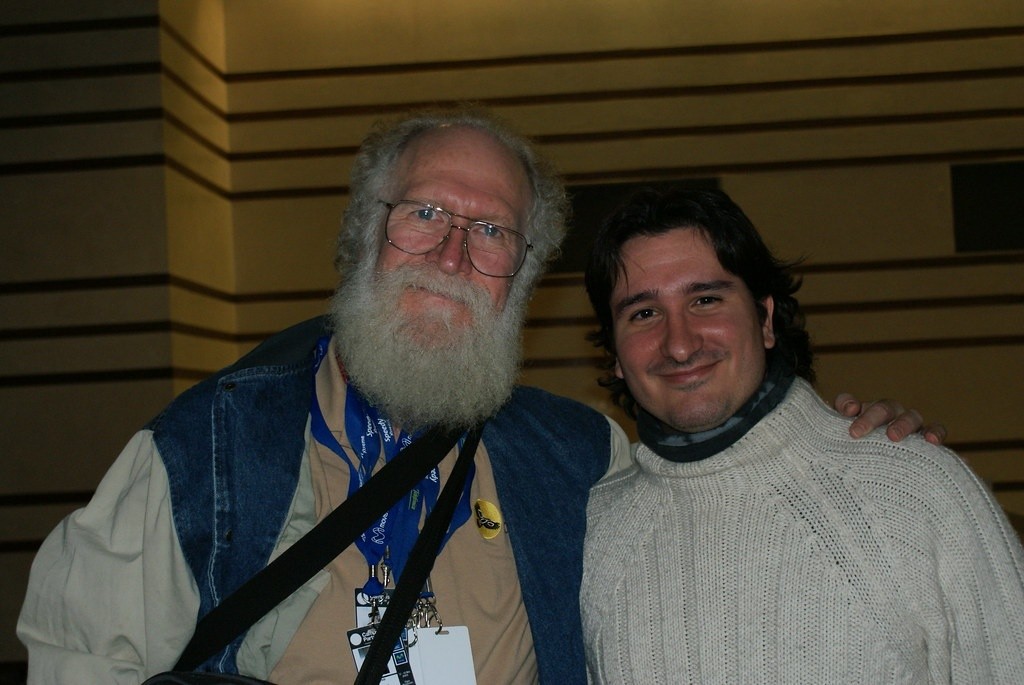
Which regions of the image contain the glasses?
[379,199,534,277]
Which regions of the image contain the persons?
[15,106,950,685]
[578,188,1024,685]
